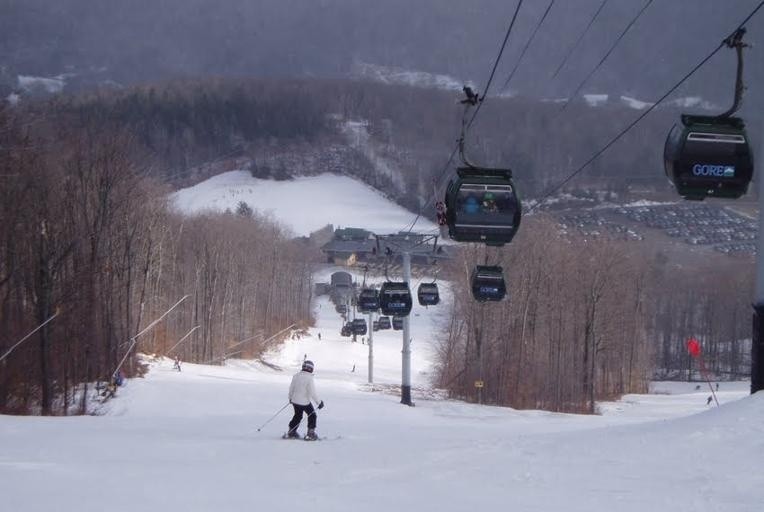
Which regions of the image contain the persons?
[351,364,355,372]
[303,354,306,360]
[361,337,364,344]
[282,360,323,438]
[172,355,182,372]
[317,333,320,339]
[366,338,369,345]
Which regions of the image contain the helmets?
[301,360,313,372]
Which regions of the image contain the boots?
[287,426,300,437]
[307,427,317,438]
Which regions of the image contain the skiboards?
[281,433,343,442]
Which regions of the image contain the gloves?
[317,400,324,410]
